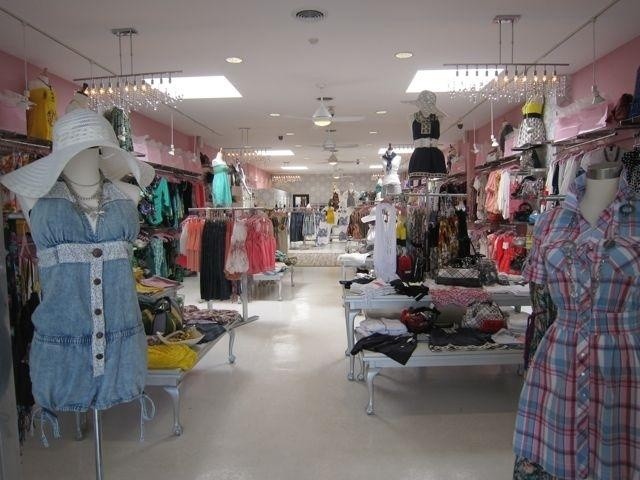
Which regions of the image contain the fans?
[283,80,364,166]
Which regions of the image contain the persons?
[25,73,58,142]
[12,144,152,418]
[496,119,514,157]
[325,205,336,244]
[380,143,402,195]
[407,90,449,180]
[234,159,246,187]
[516,91,546,148]
[342,183,360,207]
[512,161,640,480]
[446,143,458,171]
[210,150,232,206]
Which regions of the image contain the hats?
[491,312,532,346]
[1,108,157,199]
[399,90,448,119]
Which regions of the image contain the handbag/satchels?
[435,267,484,289]
[461,300,505,335]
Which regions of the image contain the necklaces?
[38,77,56,101]
[60,173,109,224]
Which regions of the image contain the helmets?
[399,305,440,334]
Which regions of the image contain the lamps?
[471,100,499,155]
[222,123,270,165]
[441,14,606,106]
[168,110,175,157]
[270,161,301,186]
[73,27,184,114]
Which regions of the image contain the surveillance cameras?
[327,108,335,118]
[457,122,463,129]
[279,136,283,140]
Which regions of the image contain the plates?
[157,329,205,348]
